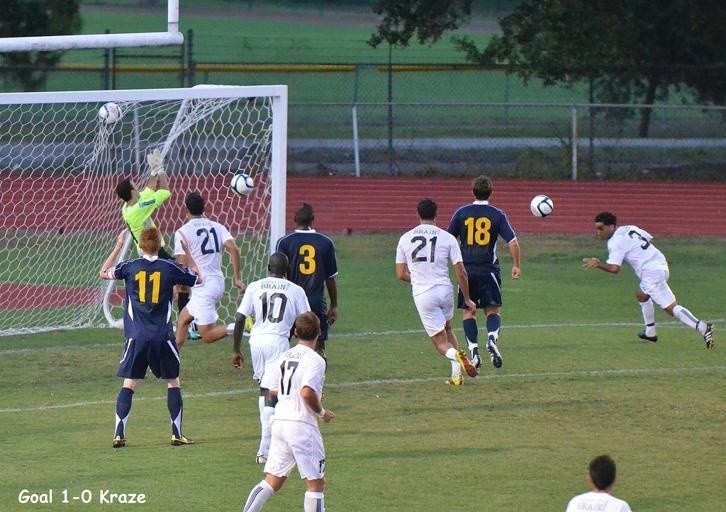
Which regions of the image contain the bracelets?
[314,407,326,418]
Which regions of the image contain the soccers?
[100,102,122,124]
[530,194,553,218]
[231,174,254,196]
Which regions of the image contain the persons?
[169,189,248,352]
[392,196,480,388]
[562,453,637,512]
[241,311,338,512]
[98,224,206,449]
[445,173,523,372]
[227,249,315,469]
[271,202,340,360]
[578,212,717,355]
[113,148,204,344]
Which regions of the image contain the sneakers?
[703,323,715,352]
[112,434,127,447]
[243,316,254,332]
[171,433,195,445]
[445,373,465,385]
[637,330,657,343]
[486,340,502,368]
[186,327,202,340]
[255,451,267,465]
[471,354,482,375]
[455,350,477,378]
